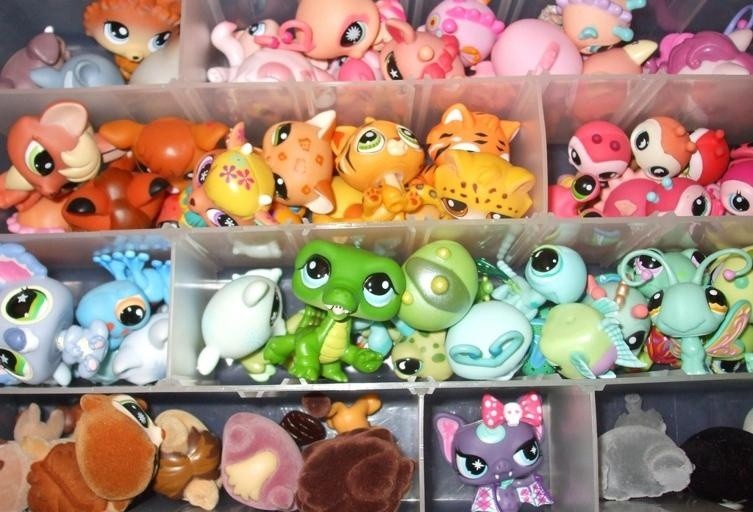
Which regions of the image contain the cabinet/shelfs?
[0,0,752,512]
